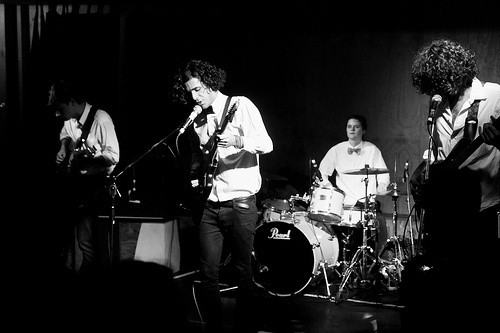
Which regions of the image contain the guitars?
[55,145,96,170]
[410,117,500,205]
[179,101,238,211]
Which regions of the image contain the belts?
[210,197,254,210]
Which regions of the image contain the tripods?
[335,166,391,305]
[366,180,411,274]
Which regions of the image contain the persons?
[317,114,390,225]
[48,80,121,267]
[169,58,273,328]
[412,39,500,310]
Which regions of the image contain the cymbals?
[262,172,290,181]
[346,169,391,175]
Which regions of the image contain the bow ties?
[348,148,361,155]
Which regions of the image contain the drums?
[260,198,307,221]
[307,185,346,223]
[250,210,340,297]
[338,205,377,229]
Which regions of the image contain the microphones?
[427,94,442,125]
[402,160,409,183]
[311,159,324,182]
[179,105,202,134]
